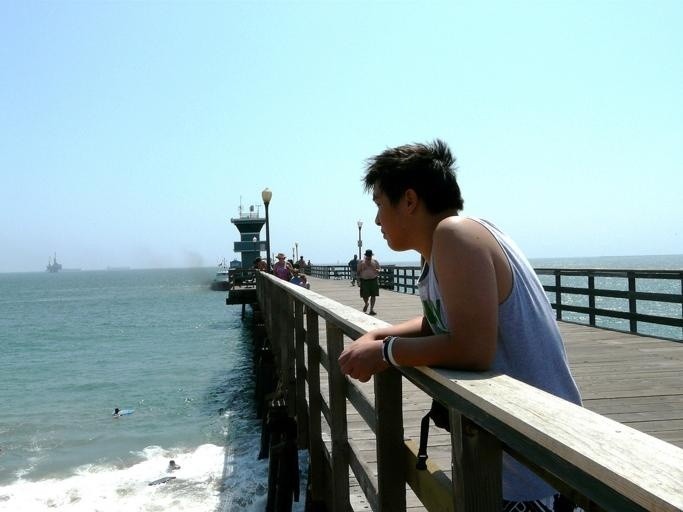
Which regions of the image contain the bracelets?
[380,334,402,369]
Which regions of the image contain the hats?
[276,254,285,259]
[364,250,373,256]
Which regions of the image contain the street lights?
[261,187,272,273]
[356,217,363,260]
[294,241,298,261]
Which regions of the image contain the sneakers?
[363,304,369,312]
[370,311,376,315]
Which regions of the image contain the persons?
[329,135,595,511]
[347,254,359,286]
[288,267,301,286]
[255,257,273,274]
[298,274,310,290]
[111,407,120,419]
[272,253,292,281]
[355,249,381,314]
[253,259,260,269]
[298,255,306,273]
[166,459,180,474]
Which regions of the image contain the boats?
[212,257,230,290]
